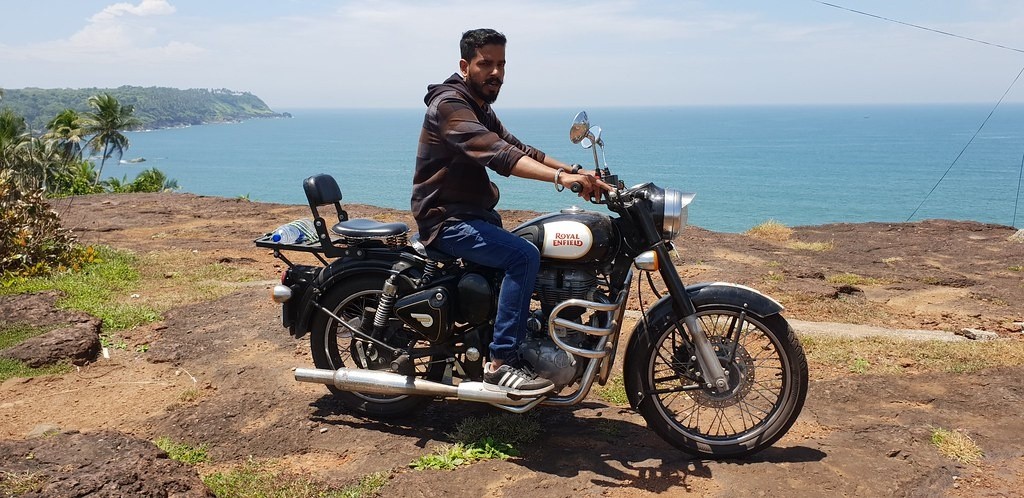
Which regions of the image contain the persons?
[411,28,614,395]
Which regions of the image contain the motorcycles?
[252,109,810,460]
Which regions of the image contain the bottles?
[272,218,314,244]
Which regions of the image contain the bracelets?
[571,163,582,174]
[555,168,565,192]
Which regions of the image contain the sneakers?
[482,359,554,395]
